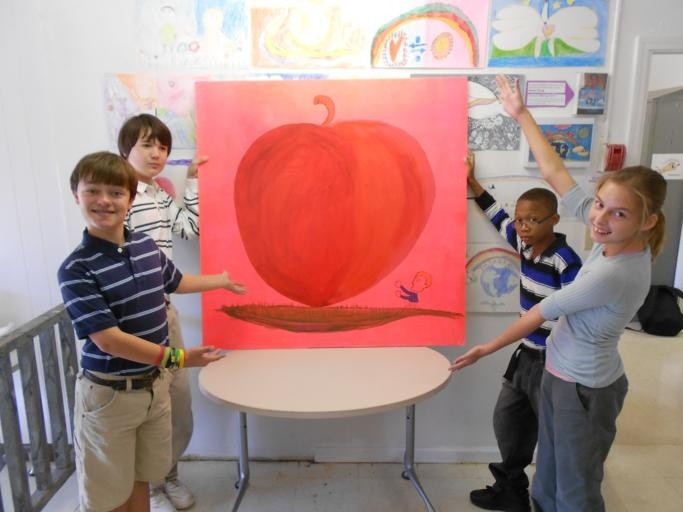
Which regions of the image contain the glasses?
[512,215,550,228]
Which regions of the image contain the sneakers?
[150,479,194,512]
[471,485,530,512]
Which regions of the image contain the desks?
[198,346,453,512]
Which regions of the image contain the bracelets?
[157,344,185,370]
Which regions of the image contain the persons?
[116,112,212,511]
[463,150,586,512]
[448,67,667,512]
[56,149,249,510]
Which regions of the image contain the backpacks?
[639,285,683,336]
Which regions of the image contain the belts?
[84,370,160,389]
